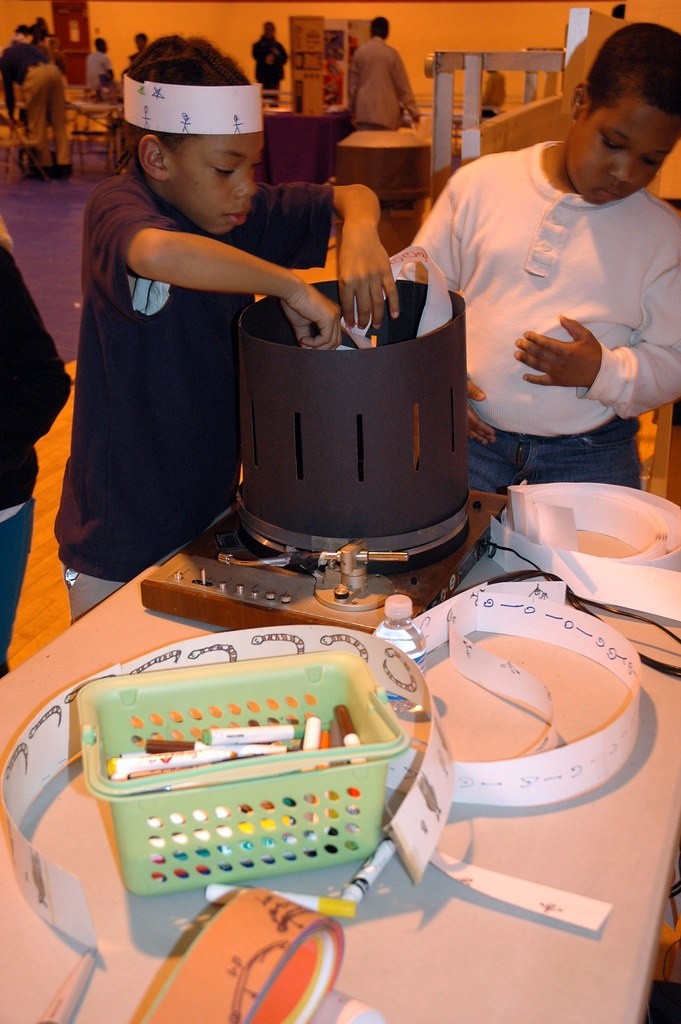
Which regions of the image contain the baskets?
[76,650,405,897]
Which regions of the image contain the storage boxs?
[76,653,409,894]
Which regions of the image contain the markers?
[206,883,358,919]
[104,705,368,881]
[340,838,397,903]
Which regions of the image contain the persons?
[0,218,76,675]
[52,37,398,626]
[251,22,288,105]
[81,30,150,104]
[0,15,152,179]
[388,21,681,493]
[345,16,427,131]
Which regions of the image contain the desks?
[1,508,681,1024]
[264,109,354,188]
[67,102,126,172]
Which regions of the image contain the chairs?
[0,114,50,184]
[68,103,116,174]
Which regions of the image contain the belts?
[25,60,50,72]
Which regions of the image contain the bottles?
[371,594,427,676]
